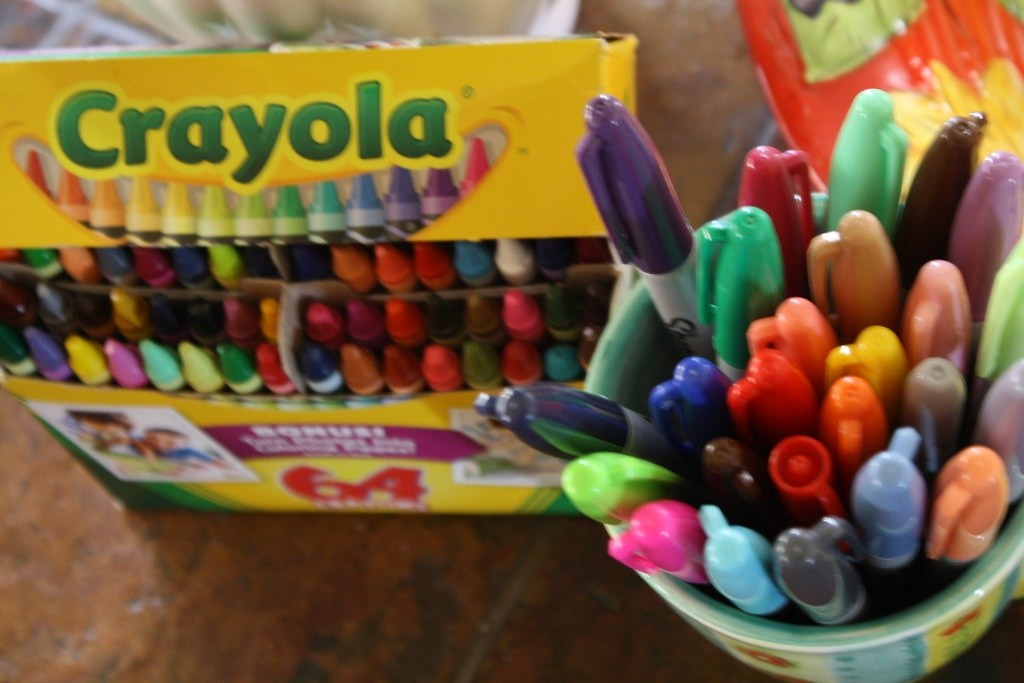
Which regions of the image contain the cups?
[583,191,1024,683]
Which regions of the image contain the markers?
[493,82,1024,631]
[0,227,606,404]
